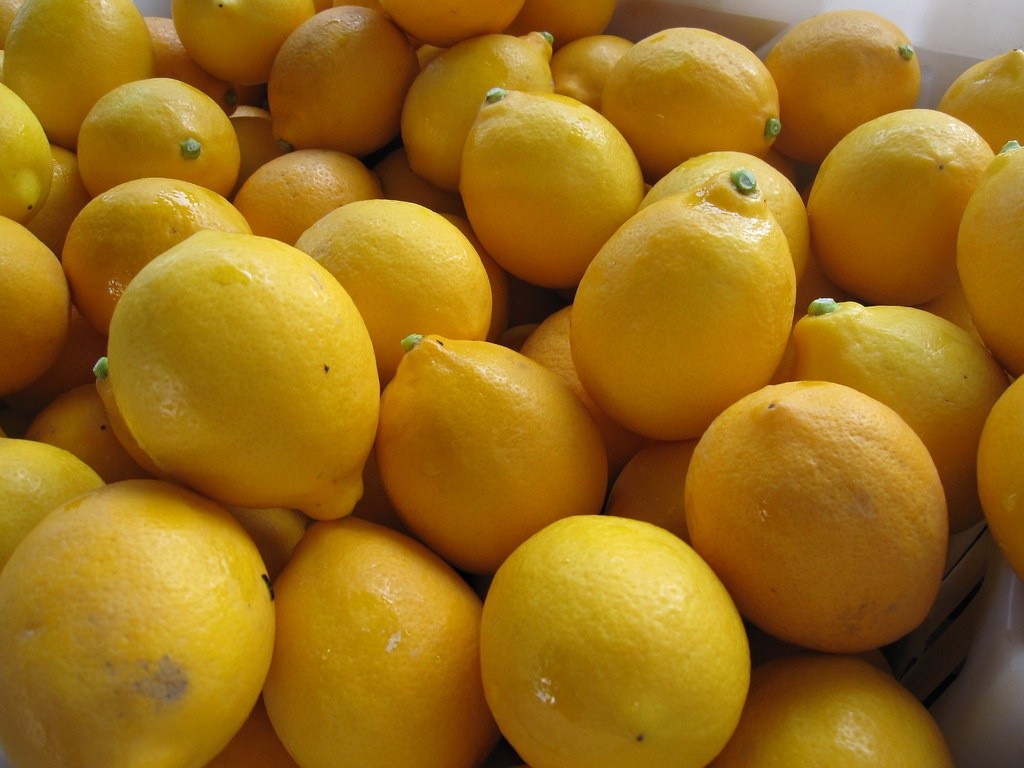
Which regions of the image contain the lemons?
[0,0,1024,768]
